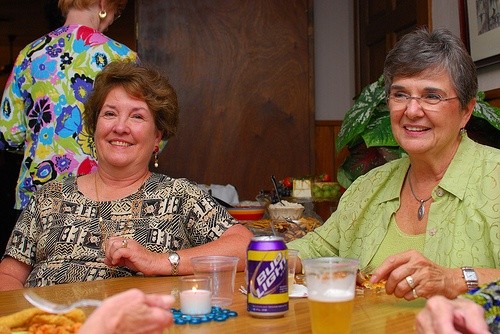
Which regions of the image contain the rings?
[412,288,418,299]
[405,276,415,289]
[122,239,128,248]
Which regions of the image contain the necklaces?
[89,170,148,260]
[408,164,432,221]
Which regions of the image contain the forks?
[22,291,103,314]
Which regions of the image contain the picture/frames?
[463,0,500,70]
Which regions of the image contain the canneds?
[246,236,290,318]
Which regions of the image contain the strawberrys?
[283,176,293,187]
[320,172,332,182]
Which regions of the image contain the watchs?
[460,267,479,293]
[166,251,181,278]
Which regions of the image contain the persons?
[71,288,178,333]
[413,278,500,334]
[0,0,168,234]
[269,26,500,301]
[0,61,258,290]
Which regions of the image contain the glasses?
[386,93,458,109]
[112,9,120,20]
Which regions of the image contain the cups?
[178,275,211,315]
[288,249,299,296]
[190,256,240,307]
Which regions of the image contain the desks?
[0,272,427,334]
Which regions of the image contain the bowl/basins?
[224,207,266,220]
[267,206,305,221]
[302,257,359,302]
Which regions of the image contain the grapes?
[311,183,342,198]
[271,183,290,205]
